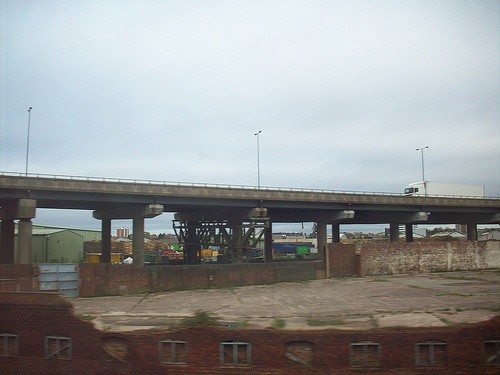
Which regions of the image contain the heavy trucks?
[404,182,485,200]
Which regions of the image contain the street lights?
[416,146,429,182]
[254,130,262,188]
[26,107,33,178]
